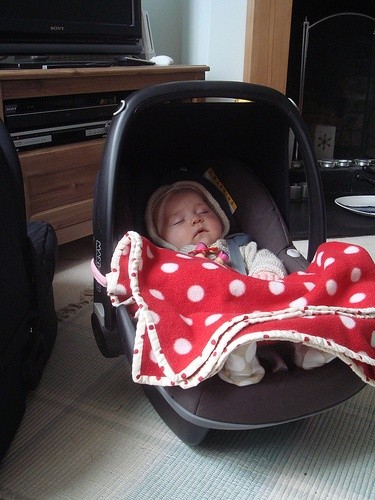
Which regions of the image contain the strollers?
[88,77,375,449]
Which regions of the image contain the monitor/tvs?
[0,0,142,68]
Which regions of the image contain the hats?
[144,179,231,251]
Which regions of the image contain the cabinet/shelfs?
[0,63,210,248]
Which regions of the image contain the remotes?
[116,57,156,66]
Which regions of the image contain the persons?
[146,185,340,386]
